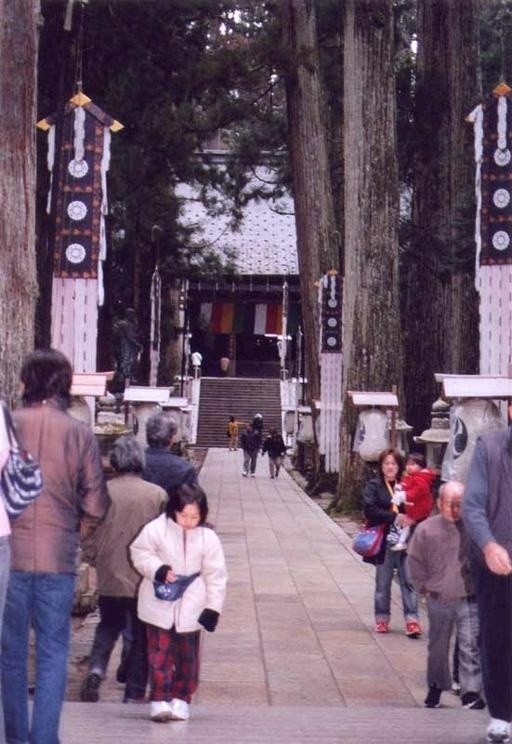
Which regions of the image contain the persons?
[361,446,421,638]
[1,347,112,744]
[220,356,231,376]
[0,390,19,620]
[389,450,434,553]
[142,412,198,502]
[460,395,512,744]
[451,633,461,692]
[80,437,166,701]
[127,481,229,724]
[227,415,249,451]
[407,479,486,711]
[252,413,264,429]
[261,425,286,479]
[190,348,202,366]
[241,421,261,477]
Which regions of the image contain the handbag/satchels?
[351,521,388,558]
[0,400,45,519]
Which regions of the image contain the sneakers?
[424,688,442,707]
[461,694,485,710]
[375,621,389,633]
[149,700,172,721]
[405,622,422,637]
[241,472,248,477]
[451,681,461,695]
[485,716,512,744]
[169,697,192,720]
[251,473,255,477]
[391,542,407,551]
[82,670,101,702]
